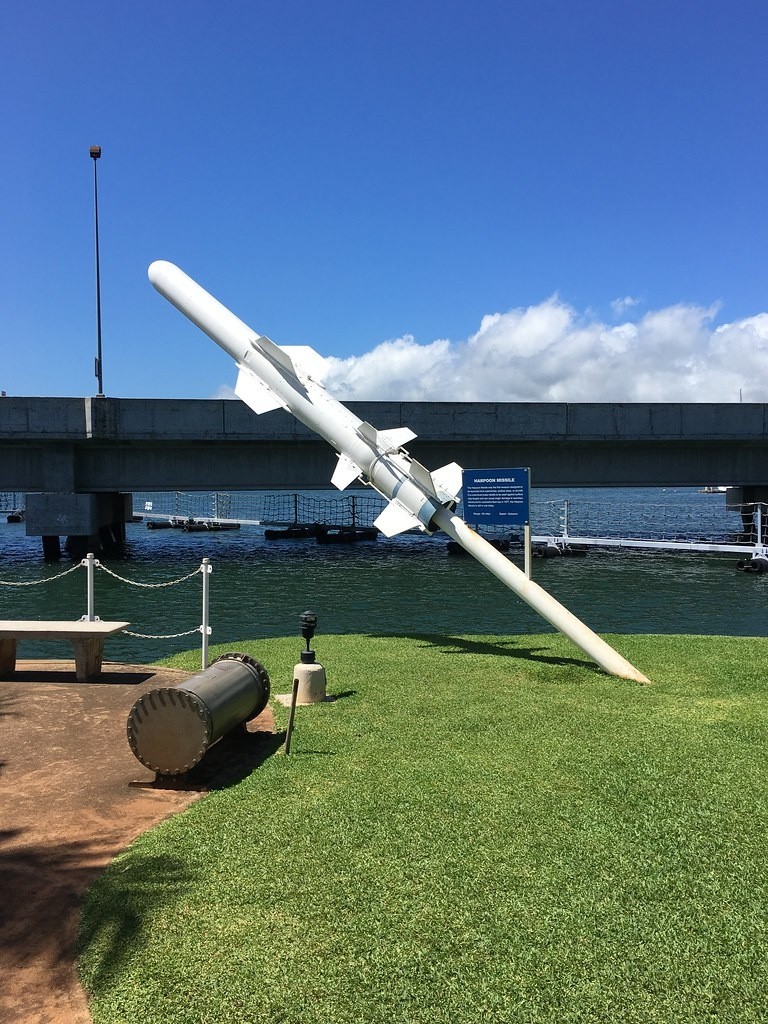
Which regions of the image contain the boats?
[308,520,382,545]
[698,486,734,495]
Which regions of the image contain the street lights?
[87,143,109,399]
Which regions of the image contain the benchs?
[0,620,130,679]
